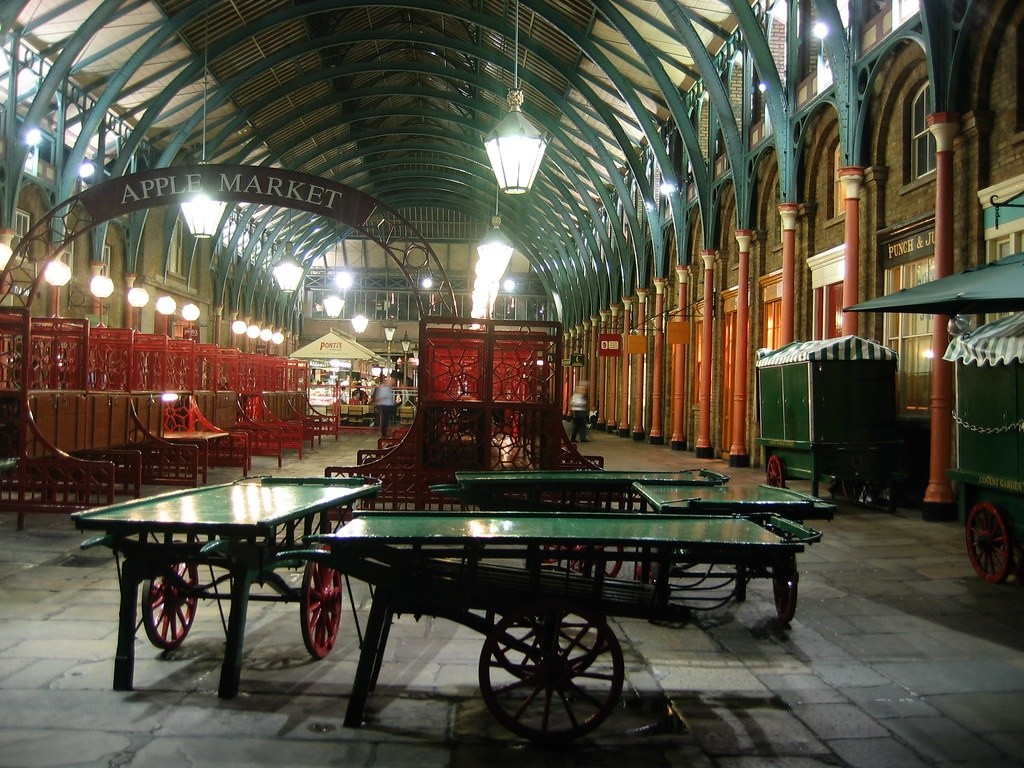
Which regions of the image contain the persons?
[341,383,369,404]
[374,377,396,438]
[571,385,591,443]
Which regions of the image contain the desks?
[73,469,832,736]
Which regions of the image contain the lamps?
[323,246,346,317]
[181,7,226,237]
[352,268,369,333]
[273,208,304,292]
[468,0,552,318]
[0,242,287,346]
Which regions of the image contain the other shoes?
[581,439,589,442]
[572,440,579,443]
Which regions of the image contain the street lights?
[381,323,397,386]
[410,344,419,386]
[400,331,411,387]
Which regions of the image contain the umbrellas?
[844,252,1024,320]
